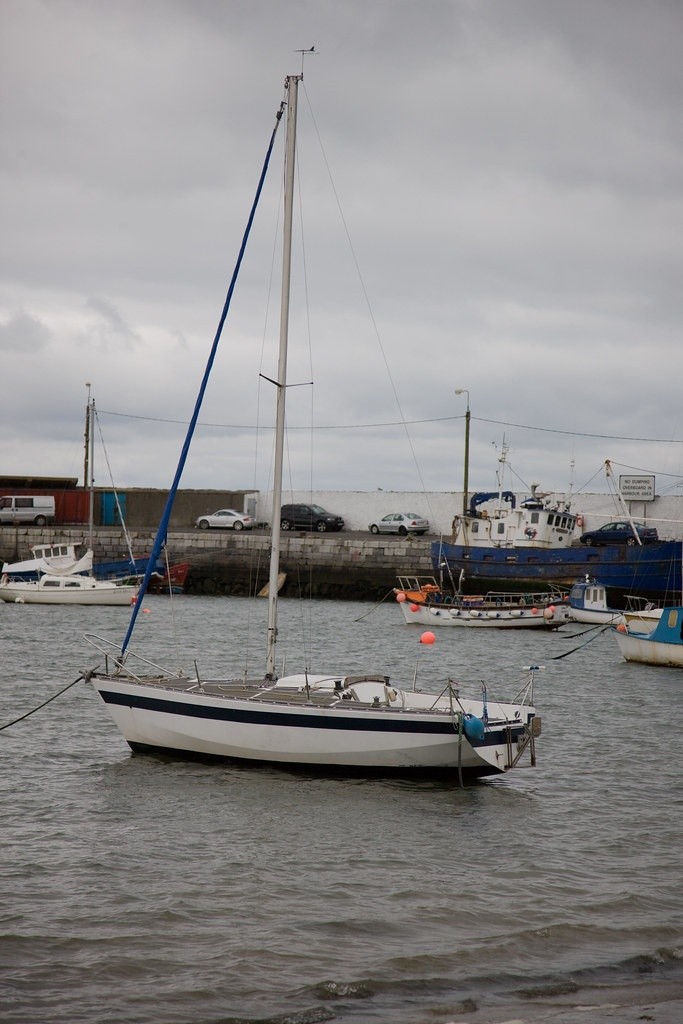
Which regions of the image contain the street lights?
[454,388,471,516]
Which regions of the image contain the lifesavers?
[525,527,536,538]
[421,586,439,592]
[576,518,583,527]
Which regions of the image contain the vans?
[0,495,56,525]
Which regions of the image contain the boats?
[550,578,633,626]
[391,574,573,630]
[145,563,189,593]
[613,595,682,668]
[431,426,683,607]
[1,541,167,592]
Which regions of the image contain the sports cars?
[195,509,258,530]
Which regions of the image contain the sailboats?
[0,399,142,606]
[88,67,542,782]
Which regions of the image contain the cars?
[279,503,344,532]
[368,512,429,536]
[580,521,659,546]
